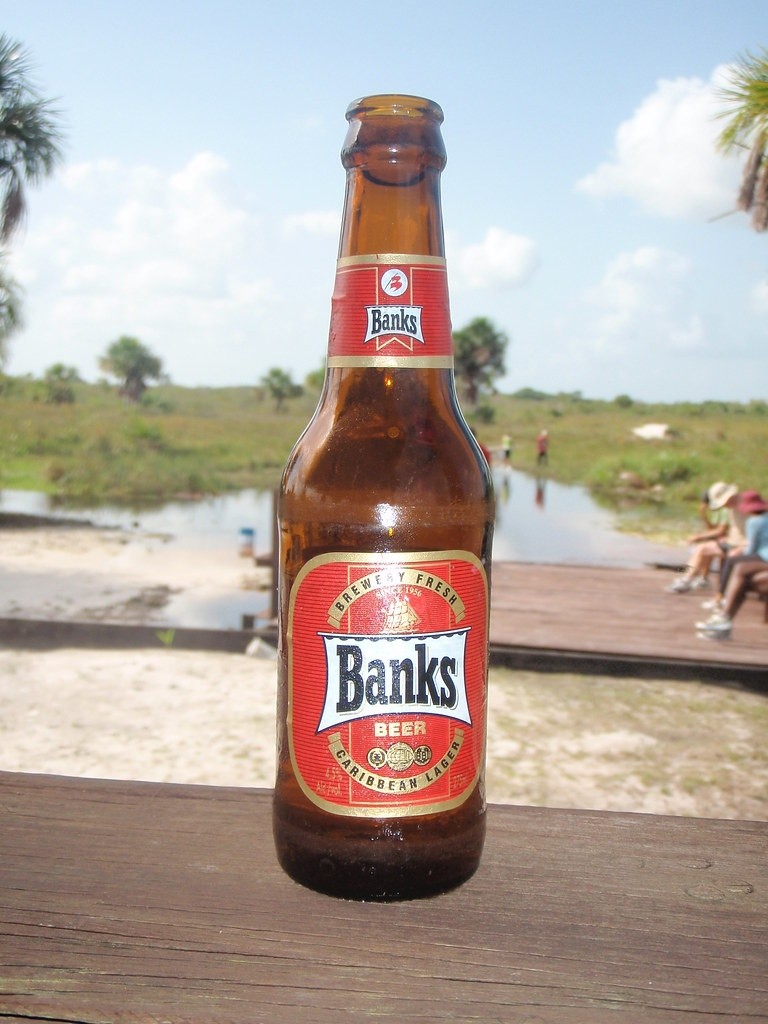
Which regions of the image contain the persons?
[701,489,729,529]
[695,562,768,640]
[664,483,747,593]
[700,491,768,612]
[236,525,259,566]
[538,431,548,464]
[501,434,513,458]
[536,479,546,508]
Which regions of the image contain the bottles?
[272,94,496,905]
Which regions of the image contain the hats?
[736,491,768,513]
[708,481,738,510]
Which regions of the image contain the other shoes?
[674,574,710,591]
[695,630,731,640]
[664,582,691,594]
[694,621,732,631]
[701,602,720,610]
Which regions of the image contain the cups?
[239,527,252,546]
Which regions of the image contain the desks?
[0,771,768,1024]
